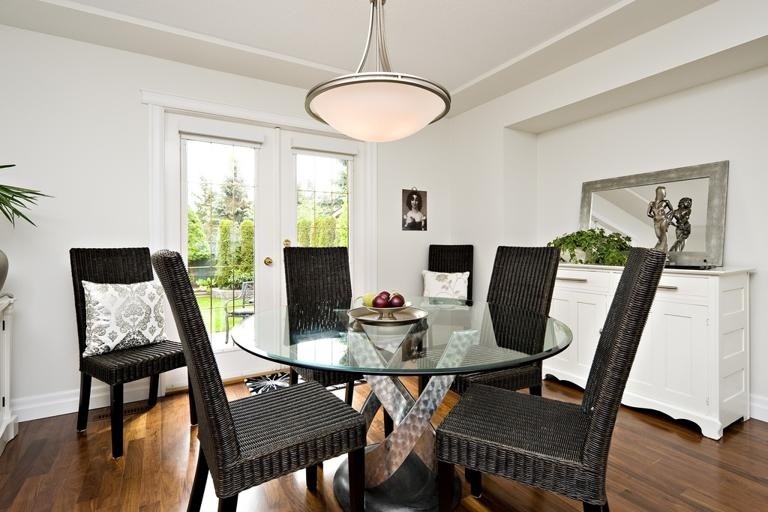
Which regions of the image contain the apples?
[363,294,375,307]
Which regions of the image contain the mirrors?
[579,159,729,270]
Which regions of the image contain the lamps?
[304,1,452,144]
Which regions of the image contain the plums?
[391,294,404,306]
[373,296,388,307]
[379,291,390,300]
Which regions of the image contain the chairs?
[433,246,666,512]
[151,249,367,512]
[224,280,255,346]
[283,246,394,438]
[428,244,474,307]
[69,247,198,460]
[418,245,561,484]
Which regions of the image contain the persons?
[669,197,692,252]
[404,191,425,229]
[646,187,673,252]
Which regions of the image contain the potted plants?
[546,226,633,267]
[0,164,57,294]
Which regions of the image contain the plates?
[362,302,412,320]
[347,308,429,322]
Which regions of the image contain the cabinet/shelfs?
[542,263,756,441]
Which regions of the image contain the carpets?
[243,370,368,396]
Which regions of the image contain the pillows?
[81,280,170,358]
[421,269,470,300]
